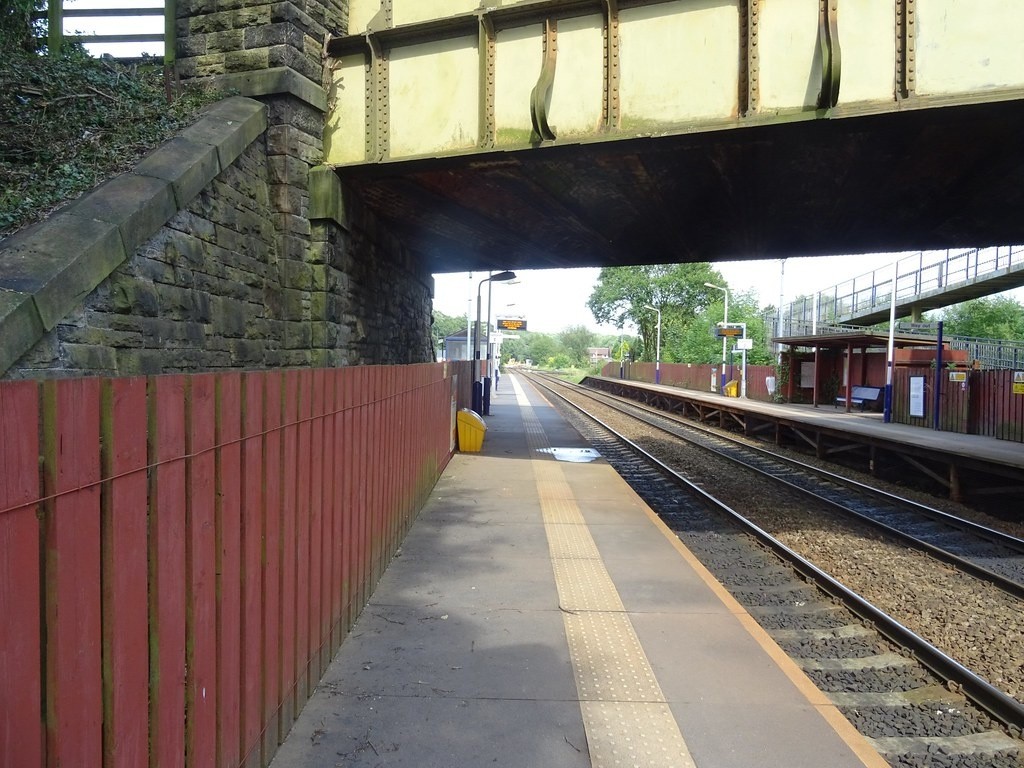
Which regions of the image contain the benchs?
[835,385,882,412]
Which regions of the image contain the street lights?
[610,318,622,379]
[475,271,516,418]
[704,283,728,396]
[644,305,659,384]
